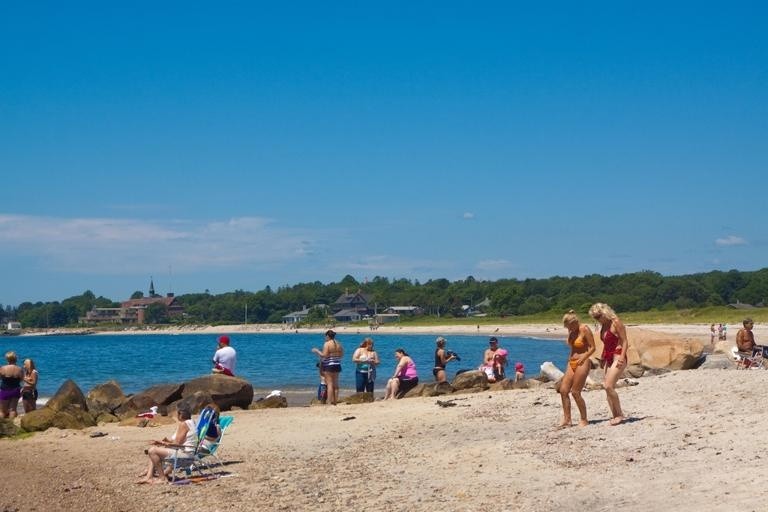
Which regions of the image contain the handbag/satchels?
[366,366,377,384]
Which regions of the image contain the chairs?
[726,345,764,370]
[145,416,235,486]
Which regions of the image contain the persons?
[0,351,24,418]
[492,348,507,376]
[484,337,505,379]
[383,349,418,400]
[433,337,457,383]
[589,302,628,425]
[212,336,237,376]
[317,359,327,404]
[312,330,343,405]
[711,323,727,343]
[558,310,596,429]
[22,359,38,413]
[139,405,221,476]
[136,409,197,484]
[352,338,380,392]
[514,363,525,383]
[736,319,767,360]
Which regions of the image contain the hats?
[217,334,230,345]
[435,336,448,347]
[489,336,497,343]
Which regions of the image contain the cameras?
[453,352,461,361]
